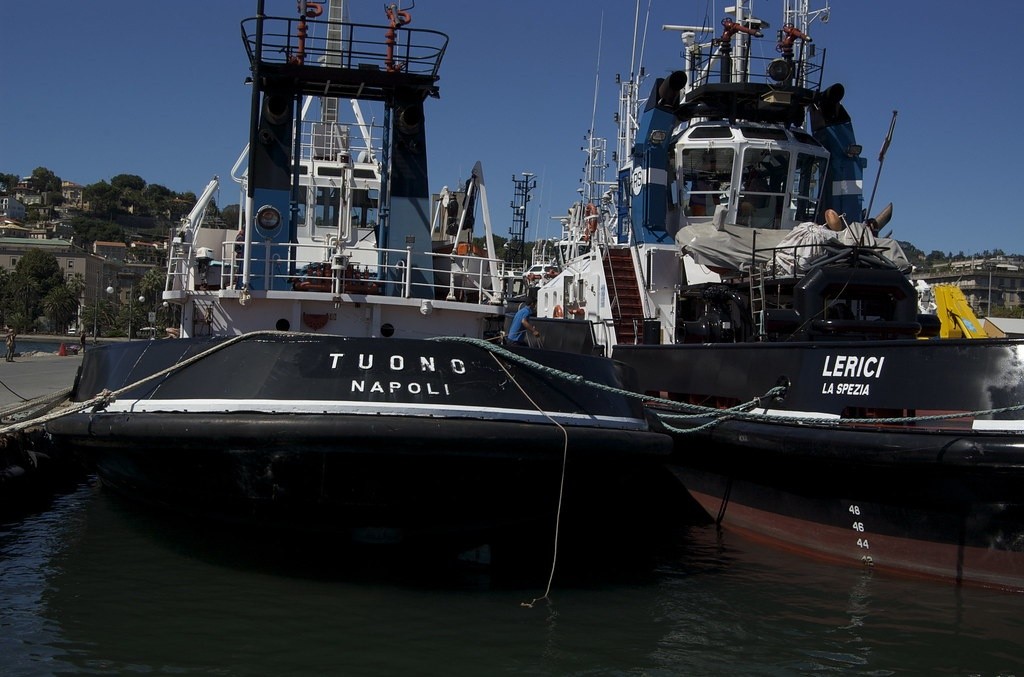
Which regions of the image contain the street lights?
[154,291,169,337]
[93,270,114,346]
[129,282,146,342]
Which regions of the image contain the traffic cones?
[58,343,67,357]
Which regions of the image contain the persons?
[78,332,87,353]
[234,224,246,290]
[6,328,16,362]
[507,296,541,342]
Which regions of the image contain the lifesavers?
[585,203,597,233]
[553,304,562,317]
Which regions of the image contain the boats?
[432,242,499,304]
[42,0,673,587]
[485,0,1024,598]
